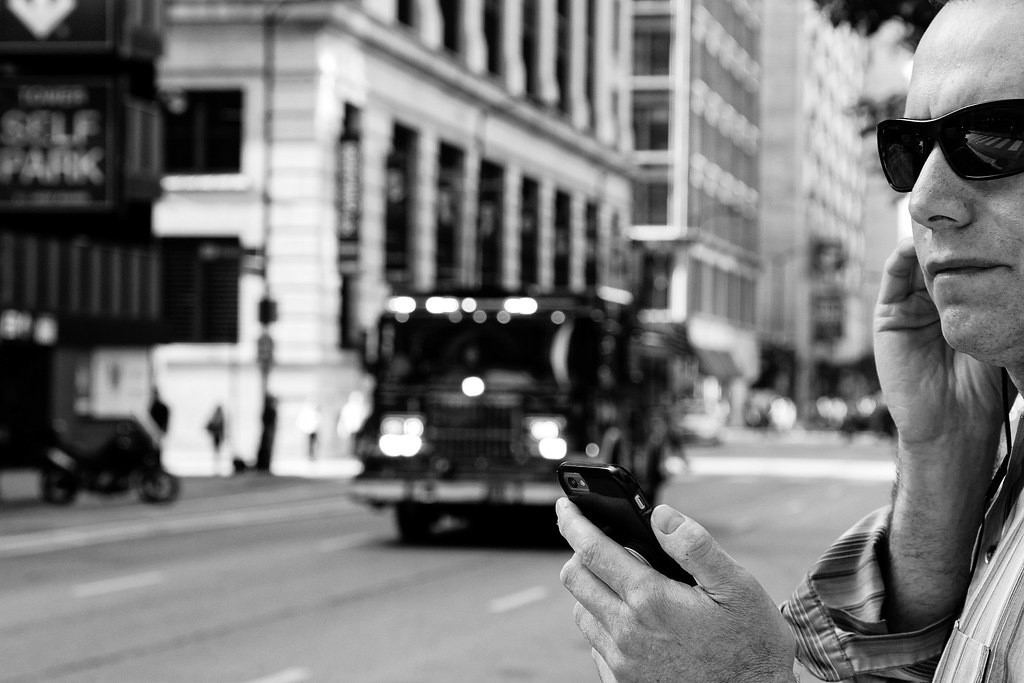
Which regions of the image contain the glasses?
[876,98,1024,192]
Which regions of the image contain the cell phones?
[556,460,698,588]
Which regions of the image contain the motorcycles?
[33,405,181,507]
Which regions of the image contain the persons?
[556,1,1023,683]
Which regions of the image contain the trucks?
[346,280,704,544]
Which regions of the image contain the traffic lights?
[258,298,275,324]
